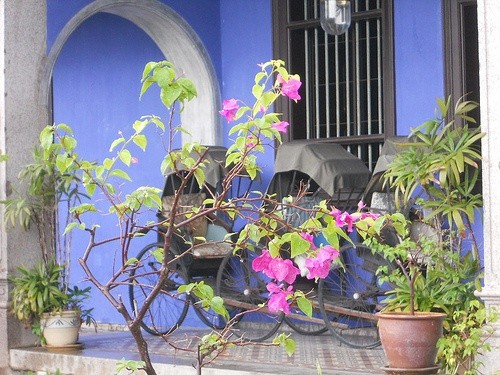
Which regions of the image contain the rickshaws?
[129,134,439,349]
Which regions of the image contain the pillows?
[276,193,319,229]
[372,191,411,238]
[162,191,210,245]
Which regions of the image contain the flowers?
[221,60,377,317]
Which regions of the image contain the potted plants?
[0,145,94,350]
[360,213,499,374]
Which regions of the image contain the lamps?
[320,0,351,36]
